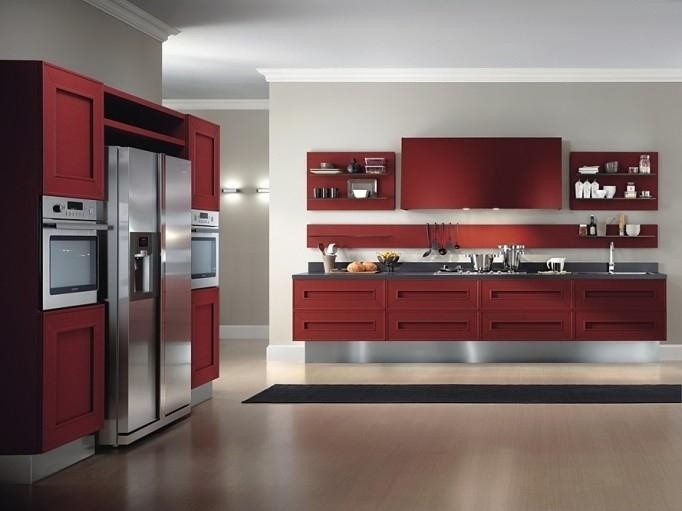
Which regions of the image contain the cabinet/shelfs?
[42,305,105,453]
[42,63,104,200]
[191,289,219,385]
[578,171,657,201]
[308,166,389,201]
[193,112,220,209]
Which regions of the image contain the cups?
[642,190,650,196]
[323,255,336,274]
[314,187,337,198]
[596,223,607,237]
[624,191,637,199]
[628,167,638,173]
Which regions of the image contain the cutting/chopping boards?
[331,270,380,274]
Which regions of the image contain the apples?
[379,251,396,259]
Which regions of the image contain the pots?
[464,252,497,272]
[497,243,526,270]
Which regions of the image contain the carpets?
[242,385,681,404]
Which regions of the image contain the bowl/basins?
[320,163,336,169]
[595,185,617,199]
[604,160,618,173]
[352,189,370,199]
[376,256,399,266]
[625,223,640,236]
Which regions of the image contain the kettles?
[546,257,567,274]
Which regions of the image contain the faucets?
[609,241,614,264]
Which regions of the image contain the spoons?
[319,243,326,256]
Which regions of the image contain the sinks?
[611,271,654,275]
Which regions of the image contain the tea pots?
[346,158,364,173]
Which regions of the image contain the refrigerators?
[92,141,194,452]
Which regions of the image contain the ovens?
[191,207,220,290]
[41,195,112,312]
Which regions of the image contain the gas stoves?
[432,264,528,275]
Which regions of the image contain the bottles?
[575,175,599,200]
[588,215,597,236]
[618,213,625,236]
[627,182,636,192]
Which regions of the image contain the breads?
[346,262,377,272]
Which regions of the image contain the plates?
[578,165,600,175]
[309,168,344,175]
[640,194,653,198]
